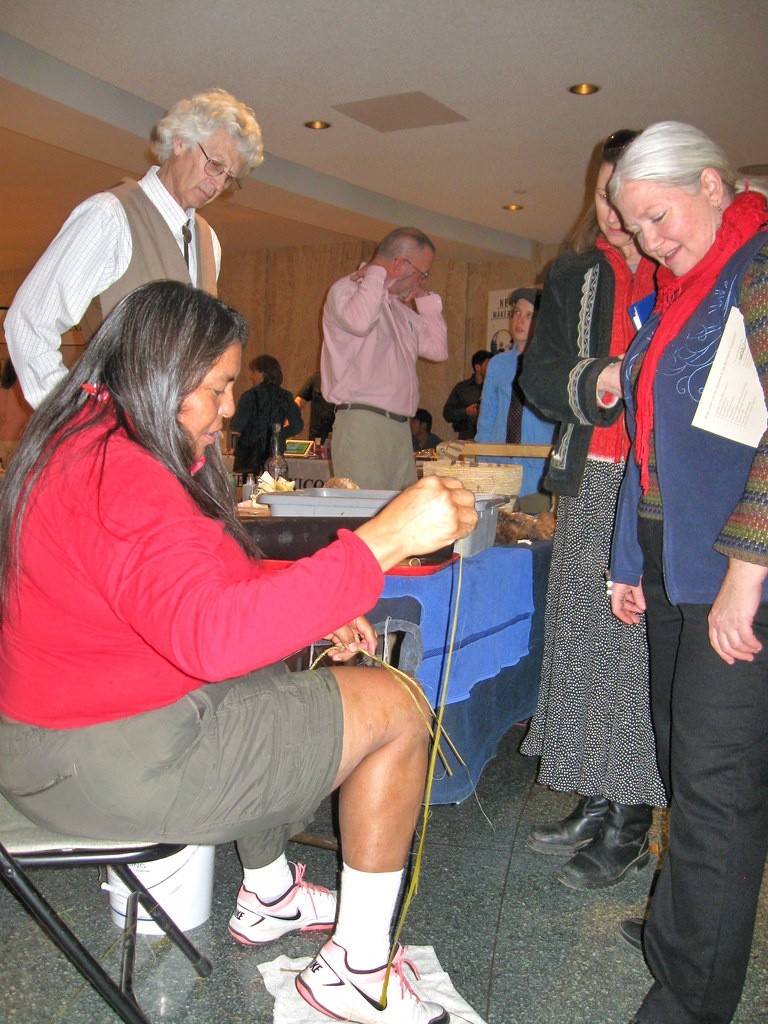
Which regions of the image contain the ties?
[506,355,526,459]
[182,220,192,272]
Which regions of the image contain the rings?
[605,580,613,595]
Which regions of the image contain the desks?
[289,538,555,854]
[221,455,334,504]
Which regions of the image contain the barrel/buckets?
[99,844,216,937]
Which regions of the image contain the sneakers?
[295,938,450,1024]
[227,861,338,946]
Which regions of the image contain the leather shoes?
[617,918,647,959]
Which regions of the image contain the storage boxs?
[258,487,510,556]
[241,517,454,564]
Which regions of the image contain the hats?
[510,288,543,307]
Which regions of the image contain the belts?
[337,404,407,422]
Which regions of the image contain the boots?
[526,795,608,856]
[558,799,651,891]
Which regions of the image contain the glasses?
[394,257,429,280]
[196,141,242,192]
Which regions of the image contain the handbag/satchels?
[232,389,263,474]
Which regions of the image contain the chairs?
[0,792,213,1024]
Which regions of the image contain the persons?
[319,228,449,491]
[518,121,767,1023]
[443,349,497,443]
[3,86,266,412]
[473,287,559,499]
[0,279,479,1024]
[410,408,443,462]
[228,354,305,485]
[293,370,335,447]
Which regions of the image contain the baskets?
[423,461,523,513]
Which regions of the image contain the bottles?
[313,438,323,460]
[242,472,256,501]
[264,422,288,481]
[323,433,331,460]
